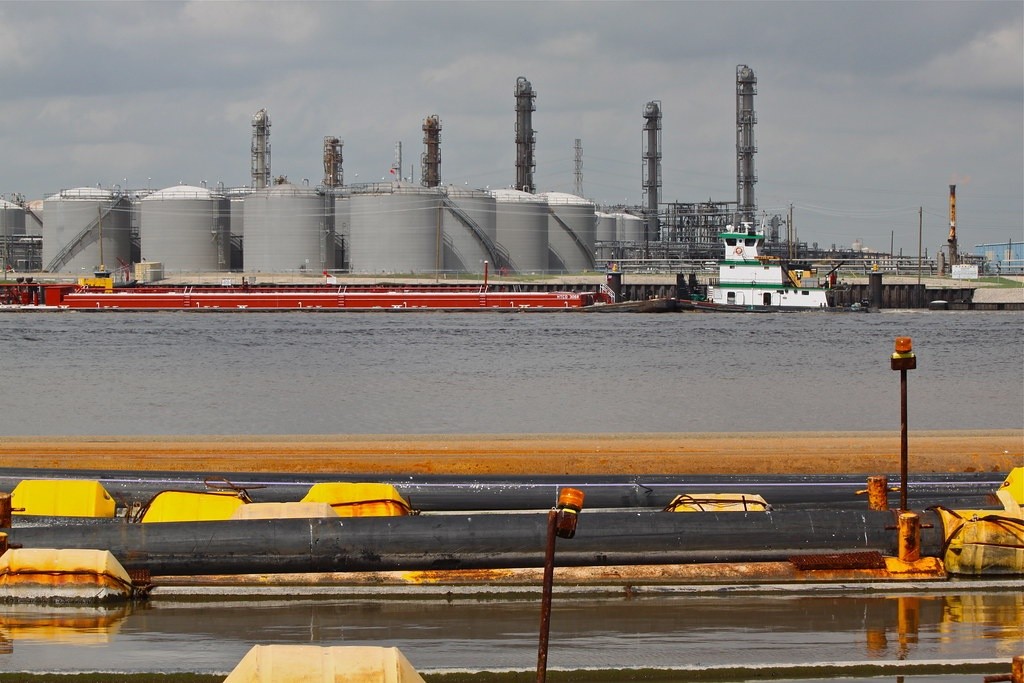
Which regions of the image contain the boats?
[705,218,881,314]
